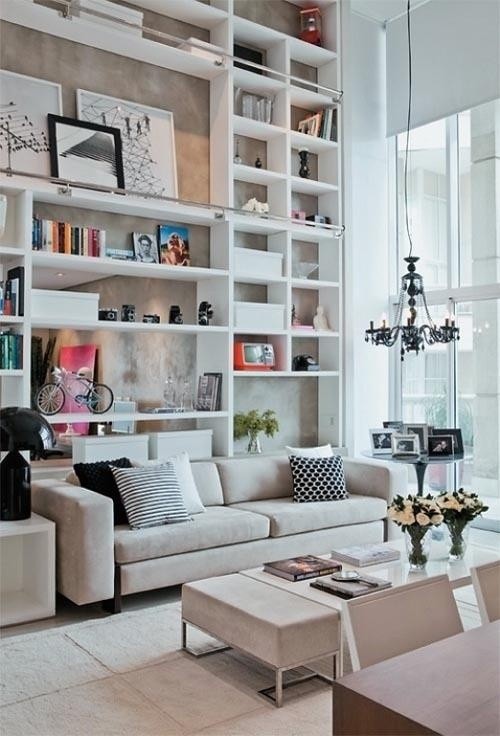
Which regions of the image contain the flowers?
[436,487,492,555]
[385,493,444,565]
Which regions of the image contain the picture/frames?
[1,68,64,183]
[368,419,466,459]
[47,113,125,197]
[75,87,179,206]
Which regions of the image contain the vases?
[444,518,470,561]
[403,523,432,569]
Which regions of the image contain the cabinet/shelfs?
[1,1,348,459]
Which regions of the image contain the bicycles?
[34,363,114,416]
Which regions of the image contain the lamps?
[298,4,324,47]
[0,404,57,523]
[359,1,468,363]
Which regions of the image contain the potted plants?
[234,408,279,455]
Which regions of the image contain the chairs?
[470,561,500,624]
[339,572,465,682]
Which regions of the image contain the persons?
[137,235,156,263]
[433,442,443,456]
[161,231,190,265]
[378,434,391,448]
[440,439,450,456]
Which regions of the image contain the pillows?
[285,443,333,460]
[289,454,350,504]
[74,448,207,533]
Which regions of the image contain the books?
[0,265,25,317]
[330,542,400,567]
[30,215,107,258]
[0,331,25,372]
[195,371,224,412]
[231,87,275,125]
[293,103,340,142]
[262,552,344,583]
[307,569,394,600]
[105,247,136,261]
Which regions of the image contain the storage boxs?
[232,300,286,334]
[71,0,144,38]
[29,287,101,323]
[233,247,285,280]
[144,427,214,463]
[55,433,151,467]
[175,36,223,65]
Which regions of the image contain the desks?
[0,513,57,629]
[331,618,499,736]
[360,449,478,496]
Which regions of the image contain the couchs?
[30,460,409,621]
